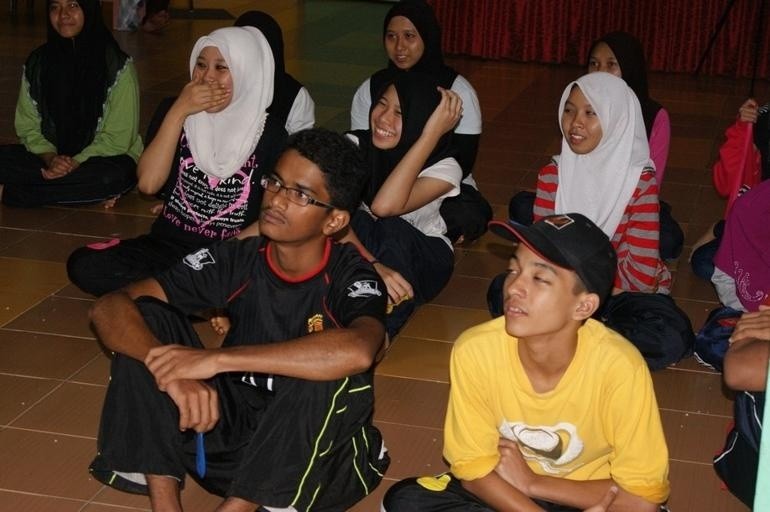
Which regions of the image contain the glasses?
[261,173,336,211]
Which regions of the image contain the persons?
[508,26,688,263]
[485,71,695,377]
[67,22,315,336]
[692,90,770,510]
[0,1,148,209]
[352,4,493,245]
[132,0,171,34]
[340,72,461,348]
[81,127,391,511]
[234,8,315,146]
[379,213,671,510]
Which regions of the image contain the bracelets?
[369,255,382,266]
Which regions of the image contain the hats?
[487,212,617,307]
